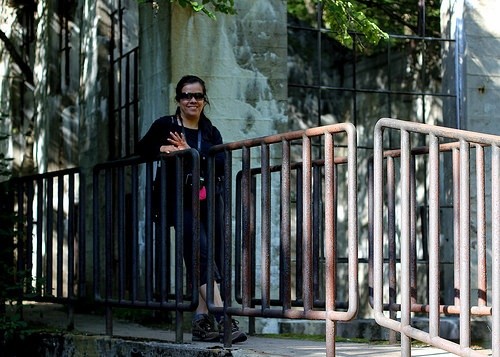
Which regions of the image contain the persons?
[135,75,248,345]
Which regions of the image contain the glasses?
[177,92,205,100]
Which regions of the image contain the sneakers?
[216,315,247,343]
[192,314,224,343]
